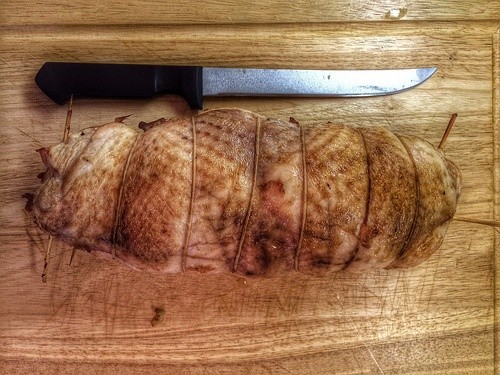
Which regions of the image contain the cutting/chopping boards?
[0,0,499,375]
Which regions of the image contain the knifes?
[35,60,438,112]
